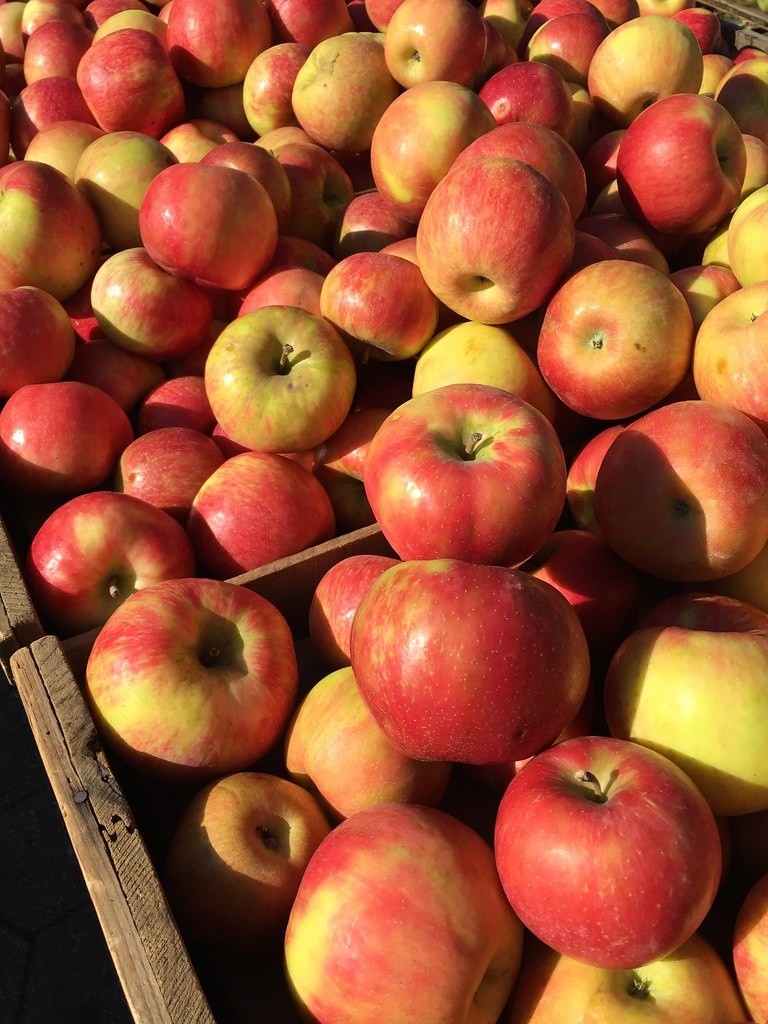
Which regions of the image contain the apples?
[0,0,768,1024]
